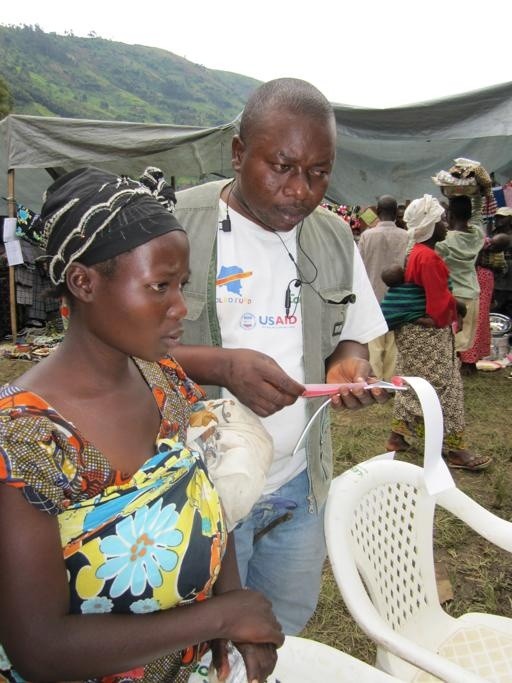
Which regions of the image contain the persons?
[166,75,392,634]
[0,161,285,683]
[357,191,512,473]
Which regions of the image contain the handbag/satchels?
[196,396,275,535]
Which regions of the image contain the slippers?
[448,452,495,473]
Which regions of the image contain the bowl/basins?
[489,312,511,336]
[439,185,484,198]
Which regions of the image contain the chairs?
[322,459,512,683]
[185,632,405,683]
